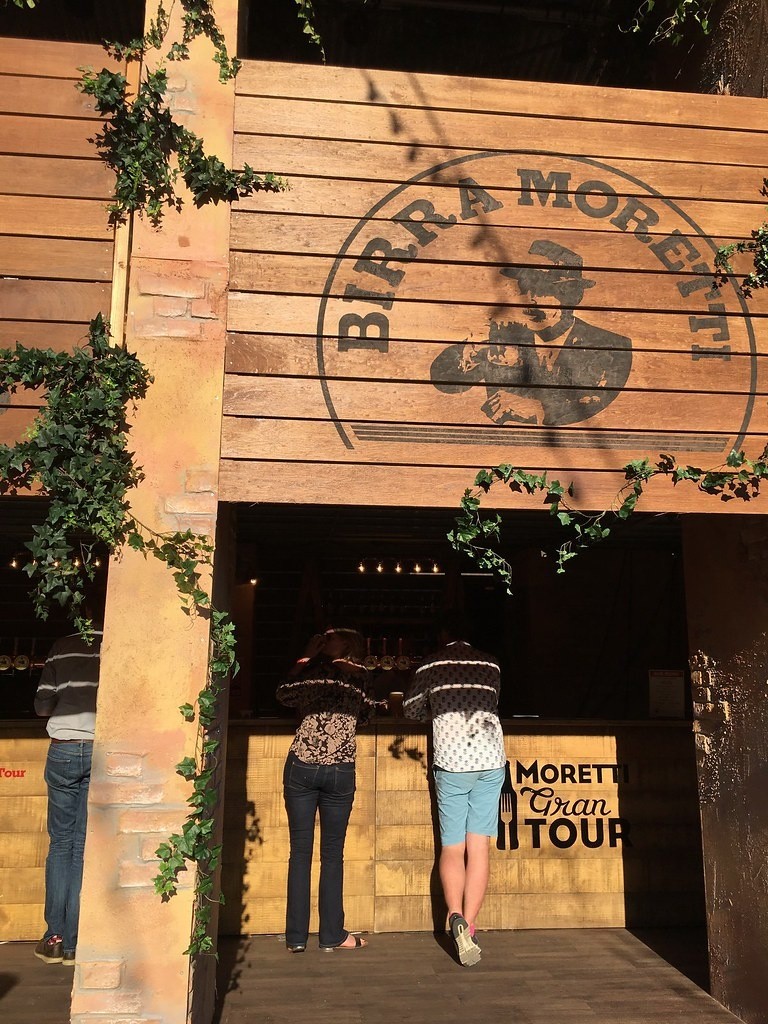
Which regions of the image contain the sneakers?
[448,911,482,967]
[62,952,75,966]
[33,938,62,963]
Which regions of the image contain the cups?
[390,694,404,719]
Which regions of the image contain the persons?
[402,609,506,968]
[34,592,106,960]
[278,628,378,952]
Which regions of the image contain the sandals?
[322,934,367,953]
[287,945,306,953]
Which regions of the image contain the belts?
[51,738,94,743]
